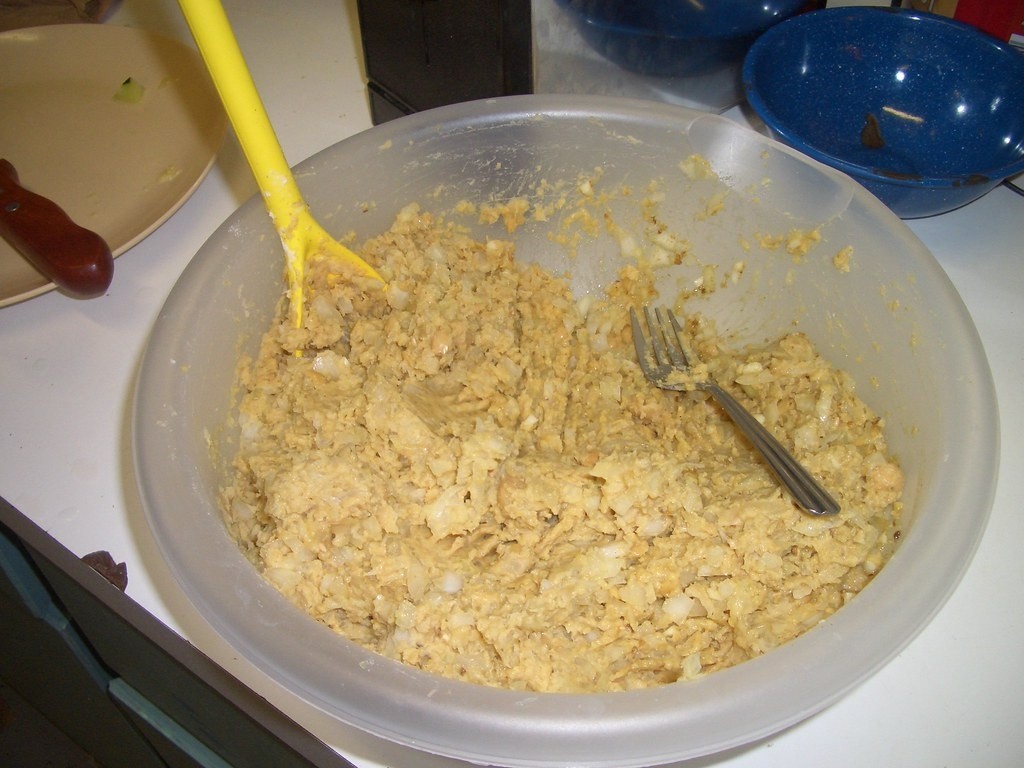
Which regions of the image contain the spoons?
[177,0,390,358]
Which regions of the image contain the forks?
[630,306,842,516]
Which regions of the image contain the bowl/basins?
[741,5,1024,222]
[130,90,1001,767]
[554,0,829,81]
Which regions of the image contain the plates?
[0,24,229,312]
